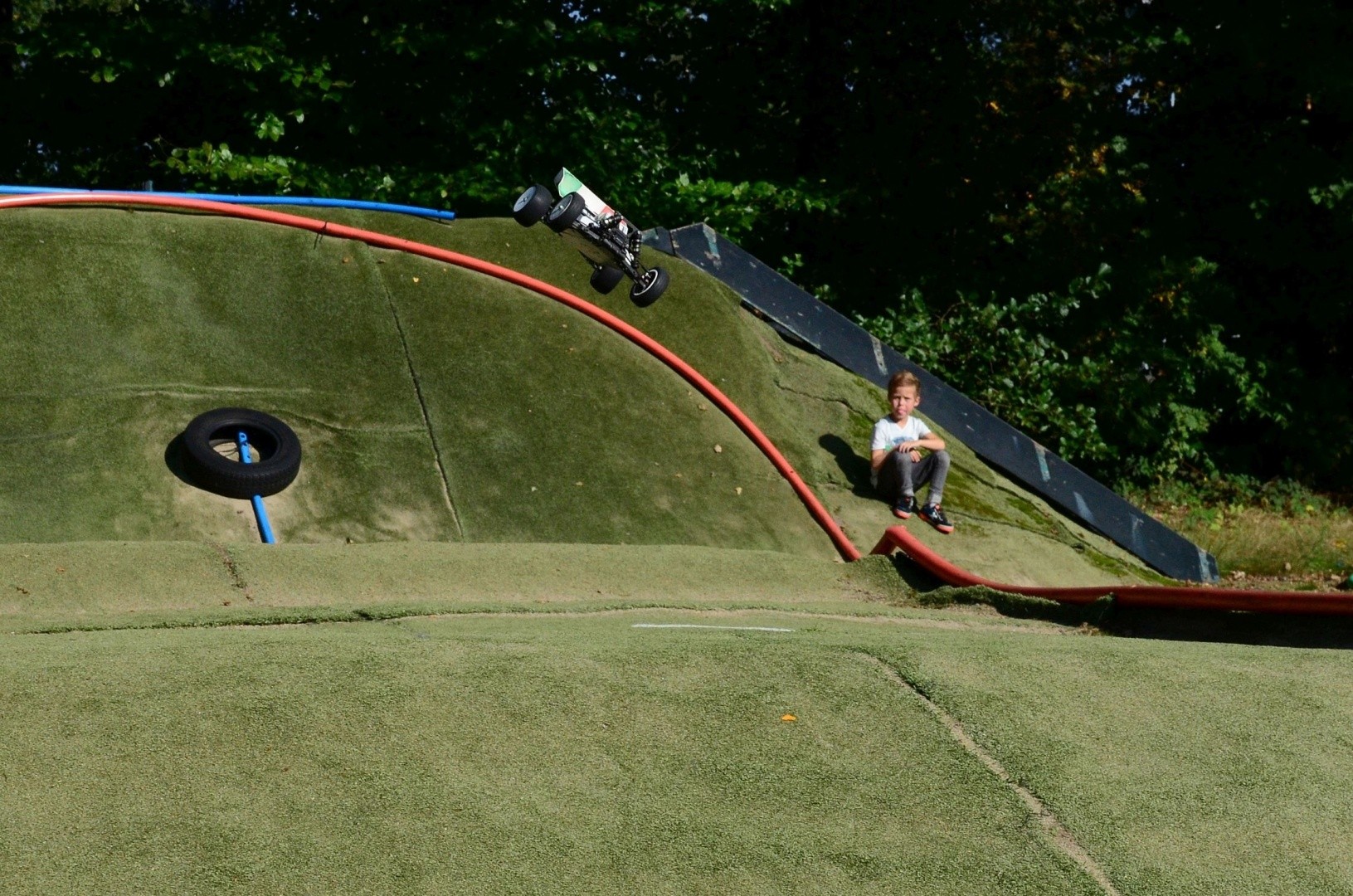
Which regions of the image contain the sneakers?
[919,503,954,533]
[895,494,917,518]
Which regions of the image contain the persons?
[870,372,954,533]
